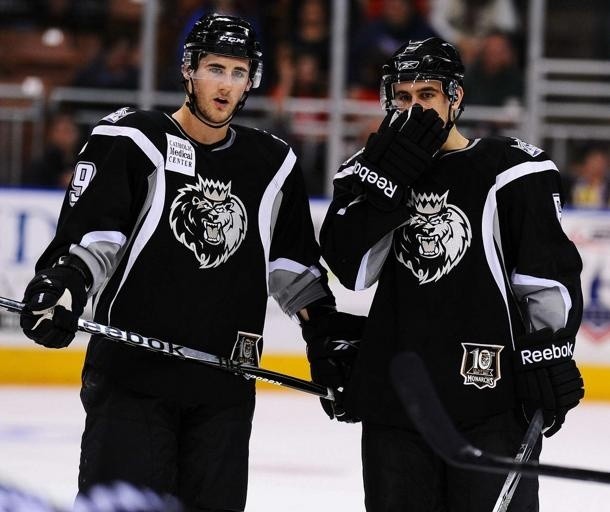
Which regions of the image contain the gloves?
[304,312,362,423]
[367,106,446,178]
[518,330,584,436]
[21,255,92,348]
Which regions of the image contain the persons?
[0,1,530,191]
[564,143,609,211]
[19,12,364,511]
[320,36,588,511]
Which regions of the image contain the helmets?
[182,15,263,83]
[383,38,463,104]
